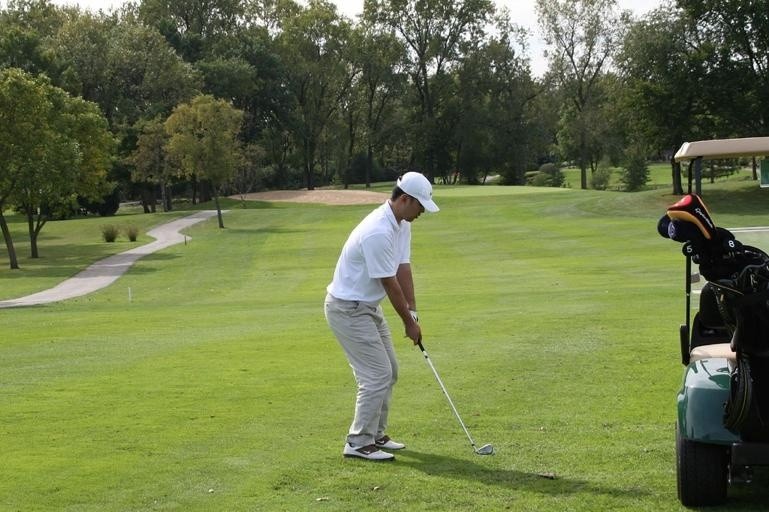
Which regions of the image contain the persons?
[324,171,440,460]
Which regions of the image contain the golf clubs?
[417,339,493,455]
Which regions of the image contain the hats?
[396,172,440,212]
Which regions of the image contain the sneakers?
[343,442,395,460]
[375,436,405,450]
